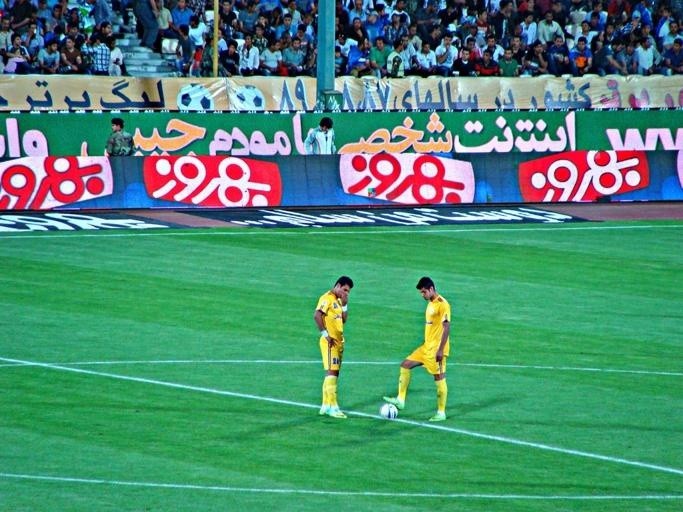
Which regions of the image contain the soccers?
[177,83,215,111]
[232,85,266,111]
[380,404,398,420]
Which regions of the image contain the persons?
[382,274,452,424]
[303,117,338,156]
[312,275,354,420]
[101,118,135,158]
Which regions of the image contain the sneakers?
[319,406,348,418]
[382,397,405,410]
[429,413,446,422]
